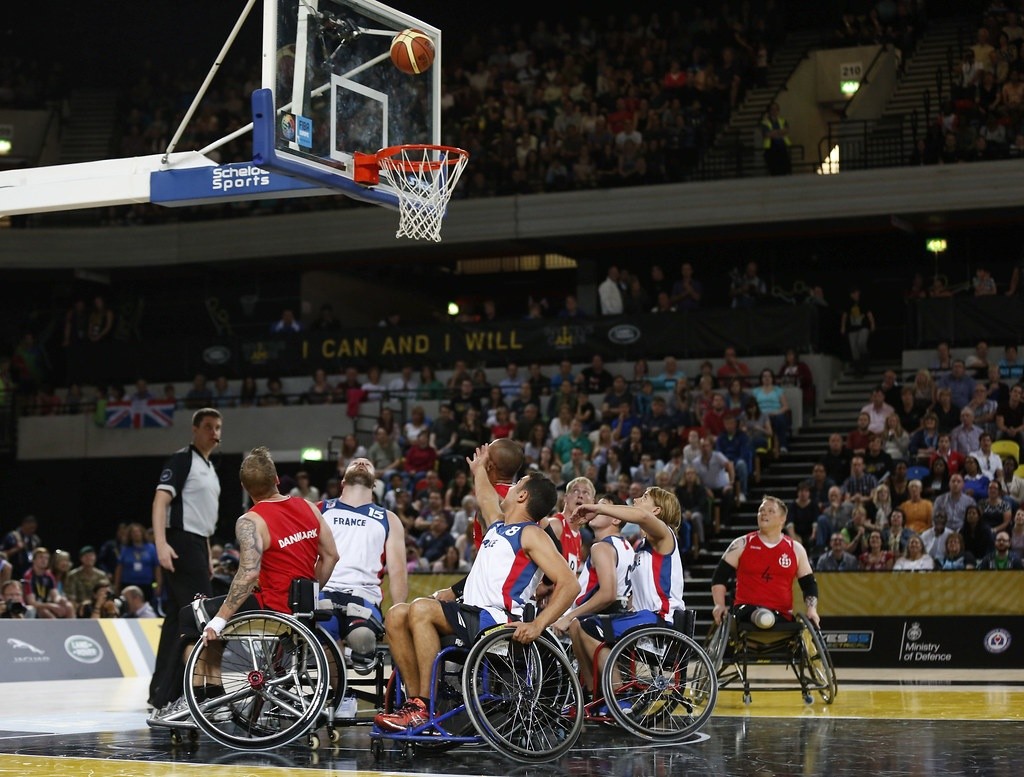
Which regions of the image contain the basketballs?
[389,26,436,75]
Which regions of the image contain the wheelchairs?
[688,604,838,707]
[148,577,350,755]
[222,592,721,768]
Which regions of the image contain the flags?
[104,401,175,429]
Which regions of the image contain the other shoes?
[561,707,569,714]
[589,694,633,715]
[205,706,233,720]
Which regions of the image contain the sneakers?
[376,698,442,735]
[334,696,357,718]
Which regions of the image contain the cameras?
[4,599,24,615]
[106,592,116,602]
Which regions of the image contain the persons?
[375,443,583,731]
[0,295,303,415]
[212,543,243,578]
[0,52,77,118]
[311,262,828,333]
[0,516,165,619]
[842,288,874,377]
[912,0,1024,165]
[712,497,822,630]
[834,0,923,47]
[434,442,686,721]
[98,1,782,226]
[312,459,407,720]
[155,448,340,721]
[785,340,1023,573]
[761,105,792,176]
[285,347,813,572]
[148,409,225,713]
[903,261,1024,304]
